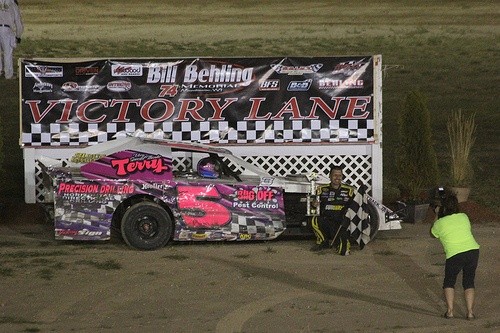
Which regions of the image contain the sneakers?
[310,243,323,251]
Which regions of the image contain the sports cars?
[53,136,402,251]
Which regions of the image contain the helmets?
[197,157,220,179]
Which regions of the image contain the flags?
[341,184,370,250]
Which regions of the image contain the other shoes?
[465,312,476,320]
[440,311,453,319]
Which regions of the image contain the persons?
[430,195,480,321]
[0,0,22,80]
[308,166,358,256]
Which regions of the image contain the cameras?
[434,187,445,216]
